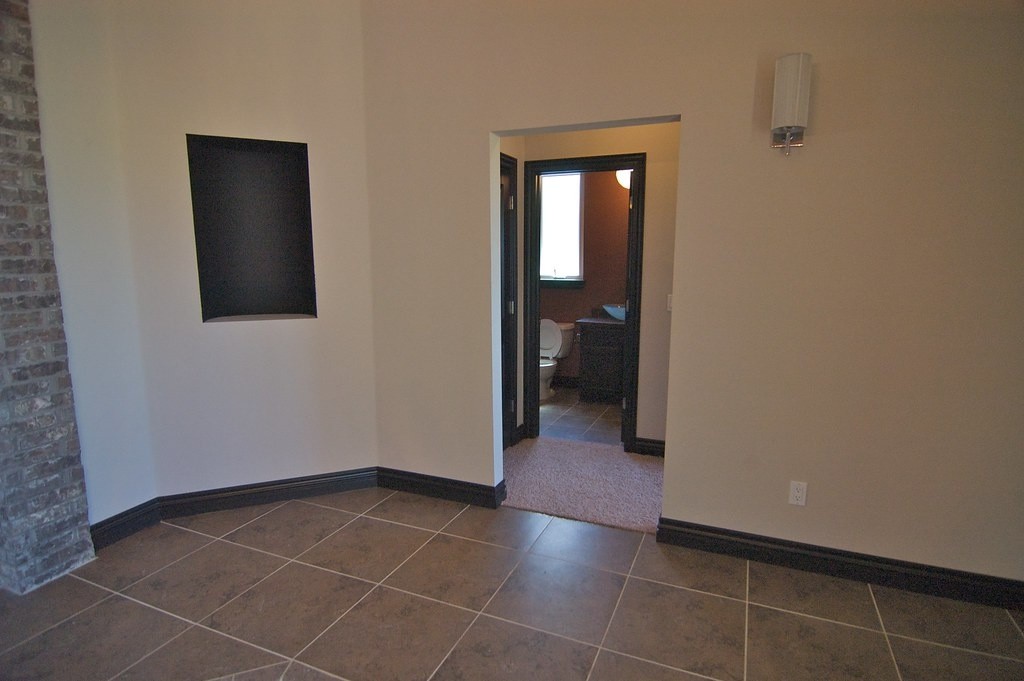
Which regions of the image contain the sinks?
[602,303,626,321]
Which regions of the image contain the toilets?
[540,318,575,400]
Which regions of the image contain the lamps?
[771,52,812,156]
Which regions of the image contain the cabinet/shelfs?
[576,317,626,405]
